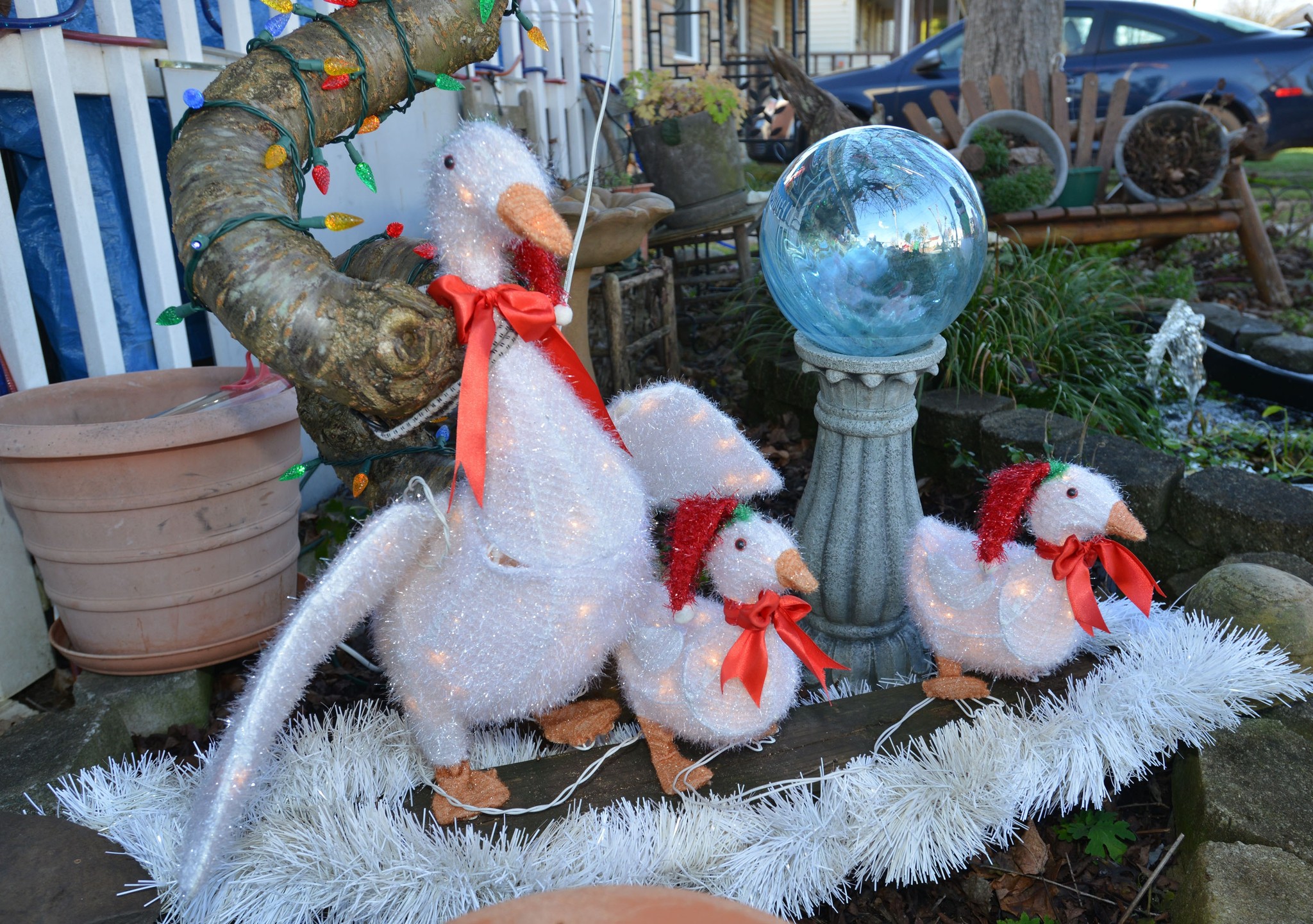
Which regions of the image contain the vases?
[1,367,300,677]
[1115,101,1233,201]
[1049,165,1104,209]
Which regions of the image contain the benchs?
[906,69,1294,311]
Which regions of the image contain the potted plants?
[951,109,1070,212]
[617,64,753,229]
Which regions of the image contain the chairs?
[582,77,768,326]
[457,79,681,393]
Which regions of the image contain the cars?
[745,0,1313,165]
